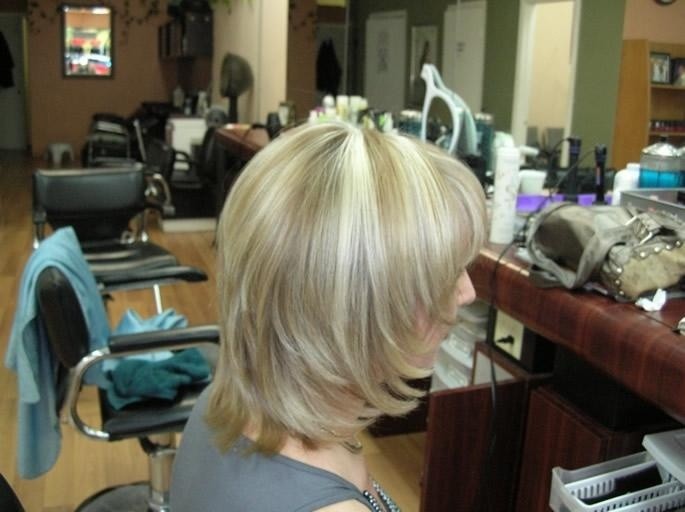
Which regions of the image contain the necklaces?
[321,426,364,454]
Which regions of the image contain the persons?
[169,120,488,512]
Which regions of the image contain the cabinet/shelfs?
[611,38,685,171]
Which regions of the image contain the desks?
[211,123,685,427]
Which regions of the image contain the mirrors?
[283,0,685,210]
[60,4,116,79]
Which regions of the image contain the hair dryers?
[251,112,281,140]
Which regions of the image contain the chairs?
[32,221,224,511]
[526,126,564,169]
[33,167,221,334]
